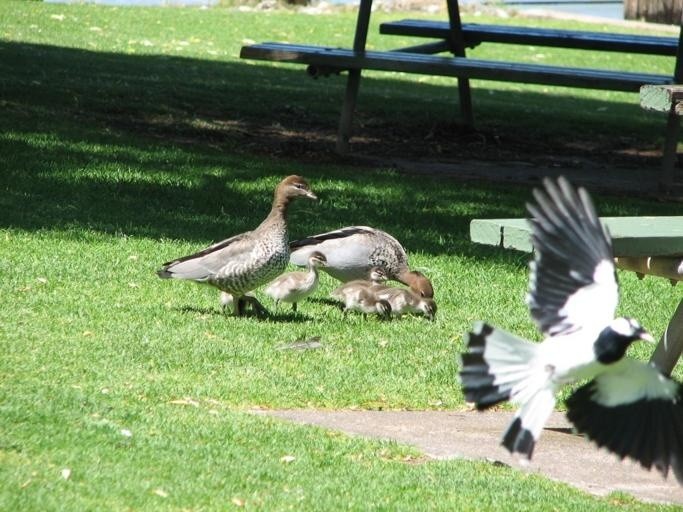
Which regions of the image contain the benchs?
[381,18,683,135]
[240,38,683,195]
[471,214,682,380]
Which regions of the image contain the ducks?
[259,225,438,323]
[153,172,318,319]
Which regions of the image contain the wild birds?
[455,173,683,490]
